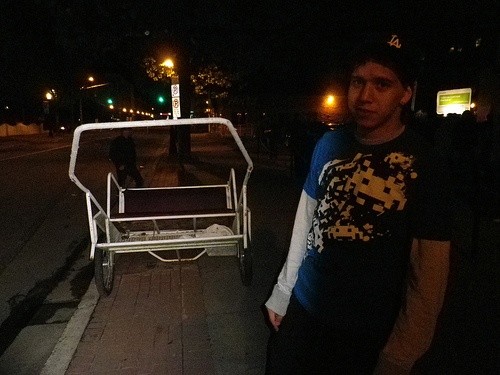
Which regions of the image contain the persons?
[109,128,145,191]
[263,28,452,375]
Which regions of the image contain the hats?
[357,33,423,86]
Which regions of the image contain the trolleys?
[67,116,253,296]
